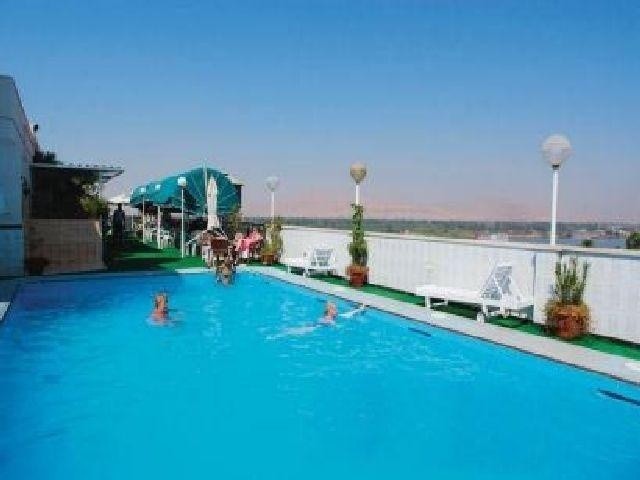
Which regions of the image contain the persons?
[217,261,231,286]
[237,224,264,262]
[265,299,370,341]
[111,204,126,250]
[144,291,185,329]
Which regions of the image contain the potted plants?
[545,249,589,340]
[260,215,283,266]
[345,202,368,287]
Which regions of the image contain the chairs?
[207,238,229,269]
[415,261,533,326]
[279,246,336,278]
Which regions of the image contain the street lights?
[541,134,572,245]
[178,176,188,258]
[141,188,147,242]
[350,163,368,205]
[155,184,162,250]
[267,175,279,227]
